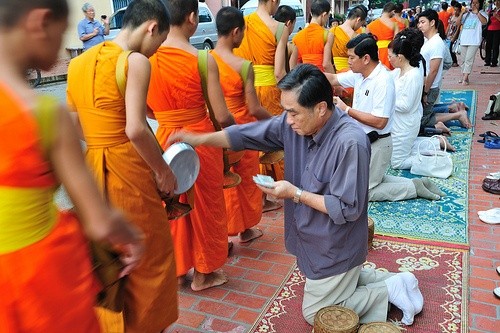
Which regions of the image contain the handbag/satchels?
[410,135,453,178]
[482,91,500,120]
[452,39,461,53]
[482,25,487,37]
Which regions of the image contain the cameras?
[468,8,473,12]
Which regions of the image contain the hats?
[82,3,90,12]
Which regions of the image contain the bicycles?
[479,38,487,60]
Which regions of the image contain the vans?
[239,0,306,44]
[102,0,218,51]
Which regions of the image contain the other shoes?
[496,266,500,276]
[484,63,491,66]
[483,178,500,183]
[459,79,469,83]
[482,182,500,194]
[490,64,498,67]
[462,79,467,85]
[478,208,500,224]
[452,63,459,67]
[493,287,500,299]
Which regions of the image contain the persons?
[208,6,272,249]
[293,0,348,98]
[0,0,143,333]
[146,0,237,290]
[324,34,447,201]
[67,0,178,333]
[168,63,423,325]
[388,36,456,169]
[328,0,500,128]
[77,3,109,51]
[275,5,296,73]
[232,0,288,212]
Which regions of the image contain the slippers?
[477,138,485,143]
[479,131,498,137]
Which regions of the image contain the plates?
[162,141,200,195]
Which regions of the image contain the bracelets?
[293,188,302,203]
[345,106,351,114]
